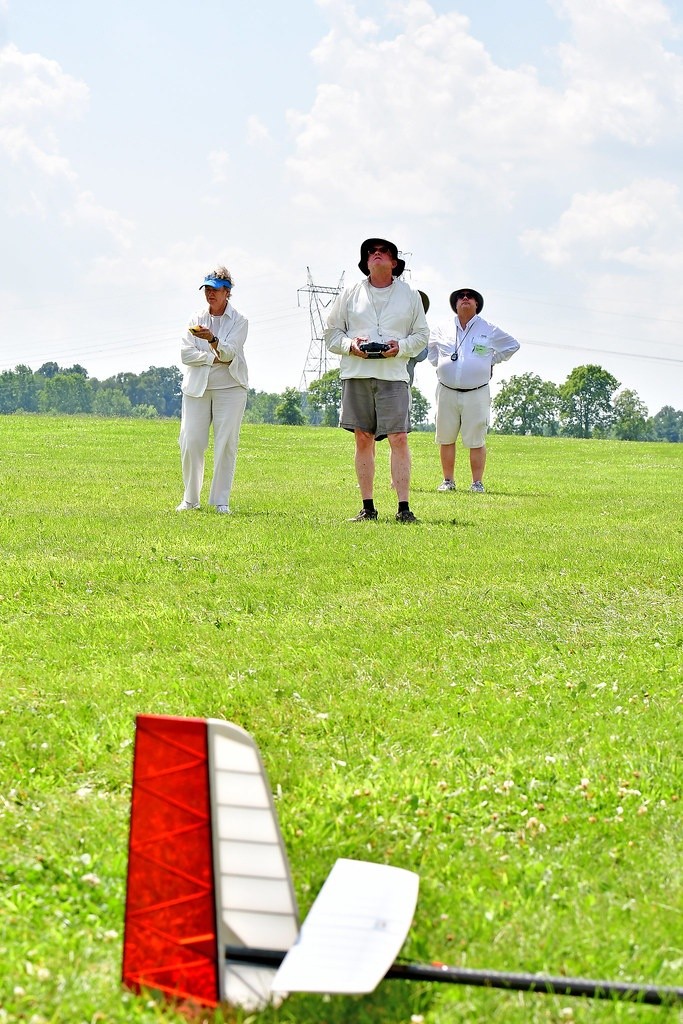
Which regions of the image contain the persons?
[428,289,521,493]
[176,267,248,514]
[323,238,430,526]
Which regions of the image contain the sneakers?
[176,500,200,511]
[217,505,230,514]
[395,511,417,523]
[347,508,377,521]
[471,481,483,493]
[437,478,455,491]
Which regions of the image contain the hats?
[358,238,405,276]
[449,288,484,314]
[198,275,232,290]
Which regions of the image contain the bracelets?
[208,337,217,343]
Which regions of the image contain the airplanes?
[116,713,682,1024]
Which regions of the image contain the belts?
[440,382,489,392]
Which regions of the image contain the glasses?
[367,246,392,256]
[456,293,477,300]
